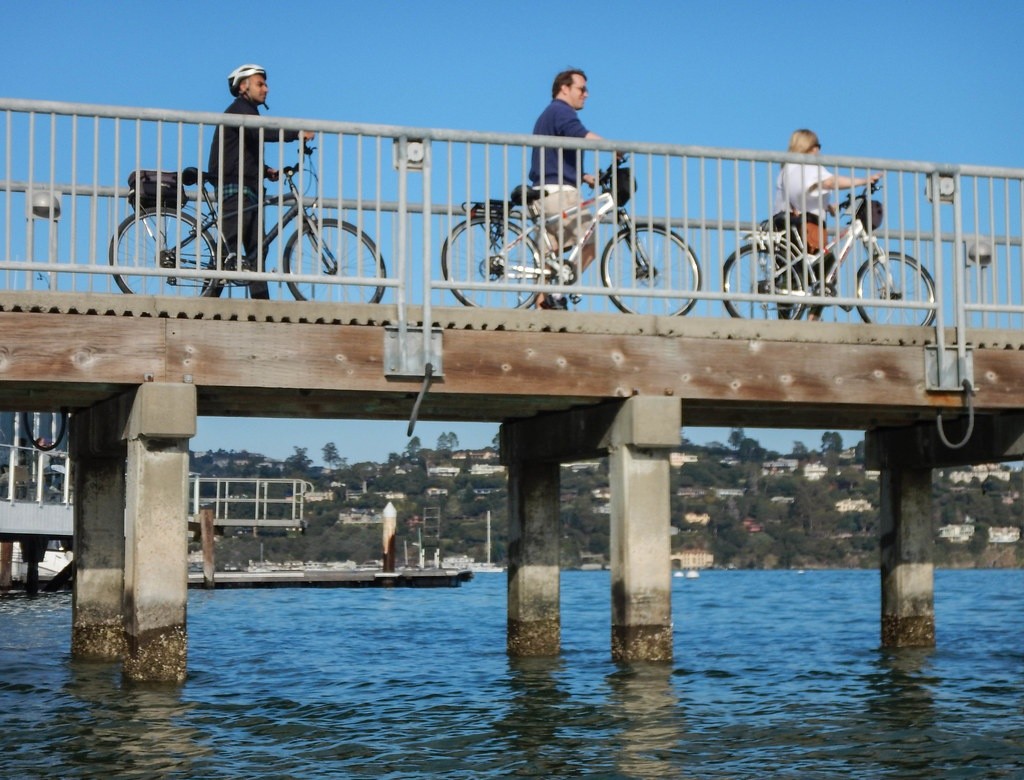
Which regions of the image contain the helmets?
[227,63,266,97]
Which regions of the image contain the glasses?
[815,143,821,148]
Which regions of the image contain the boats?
[246,543,357,571]
[440,510,503,573]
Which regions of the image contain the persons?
[201,64,315,299]
[777,129,884,321]
[528,70,624,310]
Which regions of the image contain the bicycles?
[724,180,937,327]
[108,138,386,302]
[442,149,702,318]
